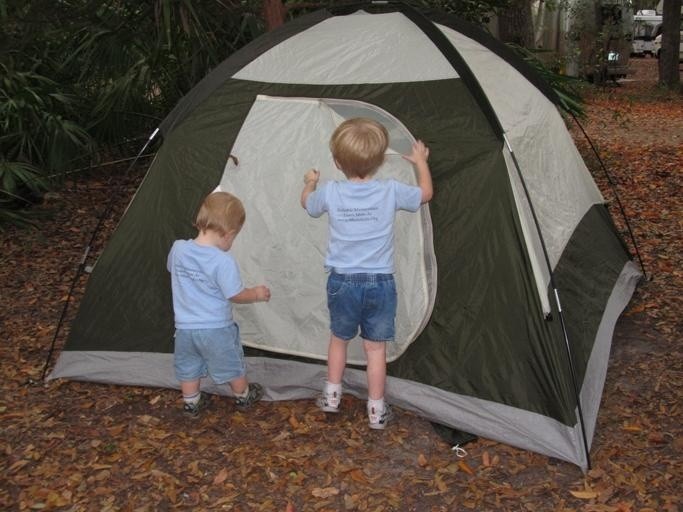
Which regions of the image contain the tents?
[42,0,653,483]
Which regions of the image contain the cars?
[605,49,628,79]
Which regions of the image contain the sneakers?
[233,382,263,410]
[368,402,392,429]
[183,392,209,418]
[315,394,341,413]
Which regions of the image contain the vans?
[631,9,683,60]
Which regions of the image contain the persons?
[167,192,271,419]
[302,117,435,430]
